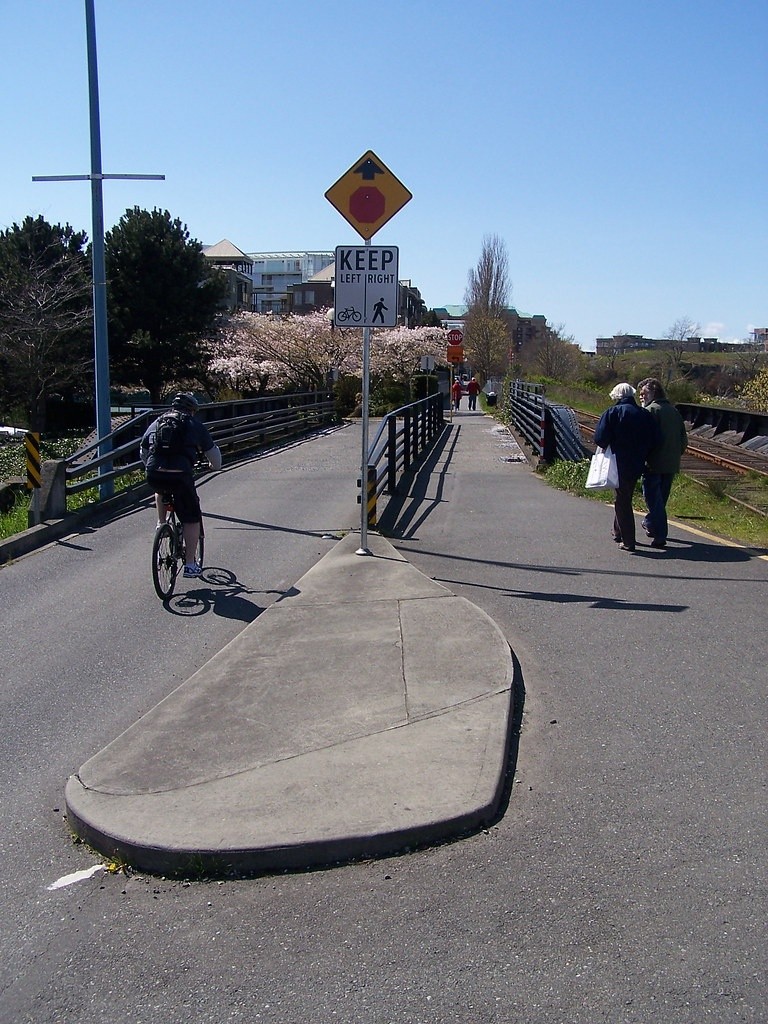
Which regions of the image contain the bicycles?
[150,461,219,602]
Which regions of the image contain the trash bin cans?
[486,393,498,406]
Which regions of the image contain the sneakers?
[156,523,161,530]
[183,566,202,578]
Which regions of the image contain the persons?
[452,380,463,410]
[638,377,688,547]
[466,377,480,410]
[594,383,652,550]
[139,394,222,576]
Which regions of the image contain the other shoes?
[618,542,635,555]
[641,518,654,537]
[651,539,666,548]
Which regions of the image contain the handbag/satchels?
[585,445,619,490]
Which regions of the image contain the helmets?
[172,393,199,417]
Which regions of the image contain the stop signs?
[447,329,464,346]
